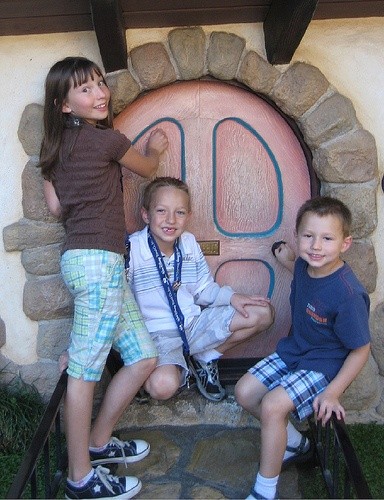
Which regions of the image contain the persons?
[234,197,371,500]
[35,57,170,500]
[58,176,275,405]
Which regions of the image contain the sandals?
[281,433,316,468]
[249,488,280,500]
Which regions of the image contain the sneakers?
[89,436,151,469]
[65,468,144,500]
[188,355,226,401]
